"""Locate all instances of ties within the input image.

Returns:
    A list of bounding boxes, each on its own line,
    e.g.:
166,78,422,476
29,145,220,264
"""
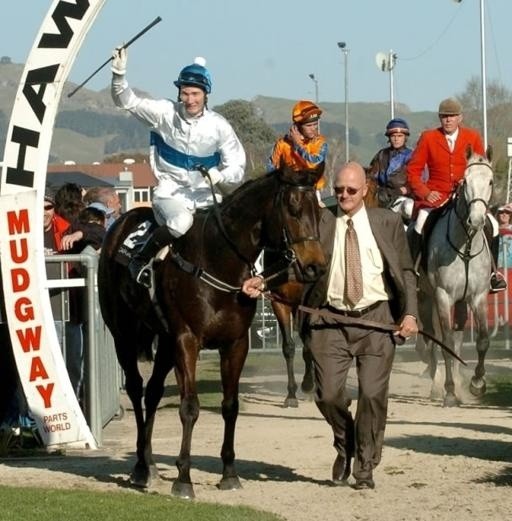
447,136,454,152
346,220,363,304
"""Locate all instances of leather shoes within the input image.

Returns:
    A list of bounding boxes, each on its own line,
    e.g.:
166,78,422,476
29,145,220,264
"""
333,455,351,482
356,479,375,490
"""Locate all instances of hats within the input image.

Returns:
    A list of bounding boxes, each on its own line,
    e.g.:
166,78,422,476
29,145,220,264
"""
88,203,114,214
45,187,55,205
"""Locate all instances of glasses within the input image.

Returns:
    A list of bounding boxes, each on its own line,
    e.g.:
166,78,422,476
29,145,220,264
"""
334,186,358,194
45,206,55,210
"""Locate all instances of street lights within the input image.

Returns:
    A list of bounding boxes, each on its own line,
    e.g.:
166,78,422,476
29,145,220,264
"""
455,0,488,148
373,47,399,124
307,71,321,135
337,41,351,162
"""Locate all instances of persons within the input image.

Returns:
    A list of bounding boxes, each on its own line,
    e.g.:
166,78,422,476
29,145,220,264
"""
10,182,121,446
241,162,417,489
367,118,427,222
270,100,327,204
407,98,506,294
496,202,512,228
111,40,246,288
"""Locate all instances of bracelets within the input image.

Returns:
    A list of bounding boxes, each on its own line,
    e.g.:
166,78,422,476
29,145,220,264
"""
405,315,416,320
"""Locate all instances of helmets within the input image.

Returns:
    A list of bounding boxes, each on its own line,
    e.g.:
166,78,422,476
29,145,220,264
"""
384,118,409,135
173,57,210,92
293,101,322,122
439,98,462,115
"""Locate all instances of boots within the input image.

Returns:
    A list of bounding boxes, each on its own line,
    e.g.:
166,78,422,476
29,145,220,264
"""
127,225,177,285
410,228,423,265
489,234,506,293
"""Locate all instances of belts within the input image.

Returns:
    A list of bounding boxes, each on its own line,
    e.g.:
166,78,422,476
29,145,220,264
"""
324,301,383,317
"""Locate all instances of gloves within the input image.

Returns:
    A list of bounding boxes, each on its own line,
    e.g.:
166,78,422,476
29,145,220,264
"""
206,168,224,185
112,40,127,75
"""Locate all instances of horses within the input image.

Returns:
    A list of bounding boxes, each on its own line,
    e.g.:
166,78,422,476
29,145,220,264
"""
348,158,437,383
408,142,497,405
98,152,325,504
262,241,317,408
